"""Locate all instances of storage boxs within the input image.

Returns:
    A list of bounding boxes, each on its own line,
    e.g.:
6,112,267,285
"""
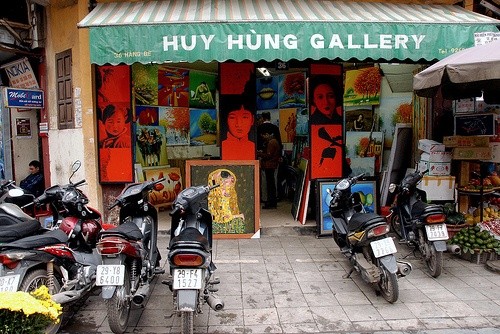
446,225,498,264
418,139,446,154
418,160,451,176
420,151,451,162
418,176,456,201
453,94,486,114
451,147,495,160
443,136,489,147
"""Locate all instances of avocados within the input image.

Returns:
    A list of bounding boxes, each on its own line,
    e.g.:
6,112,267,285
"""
447,225,500,250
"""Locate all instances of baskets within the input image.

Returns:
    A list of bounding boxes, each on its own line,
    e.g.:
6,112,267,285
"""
456,249,498,265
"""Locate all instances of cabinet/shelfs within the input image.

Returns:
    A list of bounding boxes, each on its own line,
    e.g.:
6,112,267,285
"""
457,160,500,223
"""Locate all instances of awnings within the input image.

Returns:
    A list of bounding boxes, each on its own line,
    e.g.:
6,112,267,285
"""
77,0,500,69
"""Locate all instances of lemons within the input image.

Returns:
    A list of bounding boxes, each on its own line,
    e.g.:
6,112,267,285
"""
483,207,500,222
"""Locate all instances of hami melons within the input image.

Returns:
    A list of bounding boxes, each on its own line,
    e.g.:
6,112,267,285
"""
463,207,485,224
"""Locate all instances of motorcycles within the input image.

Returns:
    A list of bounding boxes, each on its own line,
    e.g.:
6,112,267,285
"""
326,173,413,304
388,154,462,278
0,160,117,334
96,178,166,334
161,184,225,334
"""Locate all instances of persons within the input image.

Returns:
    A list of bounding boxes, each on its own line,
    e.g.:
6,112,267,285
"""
351,114,363,130
4,160,43,207
97,104,132,148
310,75,343,124
221,97,256,160
256,112,283,209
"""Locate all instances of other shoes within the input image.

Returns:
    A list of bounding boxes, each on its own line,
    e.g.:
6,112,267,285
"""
262,202,277,210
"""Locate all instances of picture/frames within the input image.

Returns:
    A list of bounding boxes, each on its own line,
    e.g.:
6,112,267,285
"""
185,158,261,240
315,177,380,240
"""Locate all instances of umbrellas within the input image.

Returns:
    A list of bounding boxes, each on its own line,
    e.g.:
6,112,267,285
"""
413,40,500,105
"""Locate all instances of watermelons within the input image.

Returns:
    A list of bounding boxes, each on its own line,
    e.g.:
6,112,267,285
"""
446,211,465,224
